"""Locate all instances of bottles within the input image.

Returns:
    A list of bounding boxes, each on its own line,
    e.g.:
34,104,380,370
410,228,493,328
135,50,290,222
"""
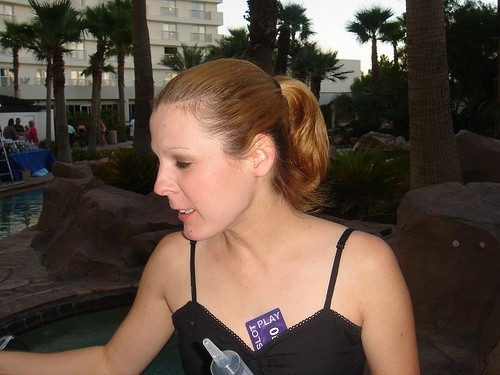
203,338,254,375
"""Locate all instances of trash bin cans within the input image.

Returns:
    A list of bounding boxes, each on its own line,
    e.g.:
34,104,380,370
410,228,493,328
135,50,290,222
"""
109,130,117,144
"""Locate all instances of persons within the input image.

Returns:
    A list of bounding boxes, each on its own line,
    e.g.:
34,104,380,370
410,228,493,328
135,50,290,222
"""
97,119,108,146
14,117,24,134
3,118,18,138
78,123,87,147
129,114,135,144
25,120,38,145
68,124,76,145
0,57,421,374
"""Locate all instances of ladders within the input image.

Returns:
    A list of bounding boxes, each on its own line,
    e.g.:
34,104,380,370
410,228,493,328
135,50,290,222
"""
0,133,14,182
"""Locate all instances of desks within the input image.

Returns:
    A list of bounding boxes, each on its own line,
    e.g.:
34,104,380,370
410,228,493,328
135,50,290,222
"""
7,148,56,180
